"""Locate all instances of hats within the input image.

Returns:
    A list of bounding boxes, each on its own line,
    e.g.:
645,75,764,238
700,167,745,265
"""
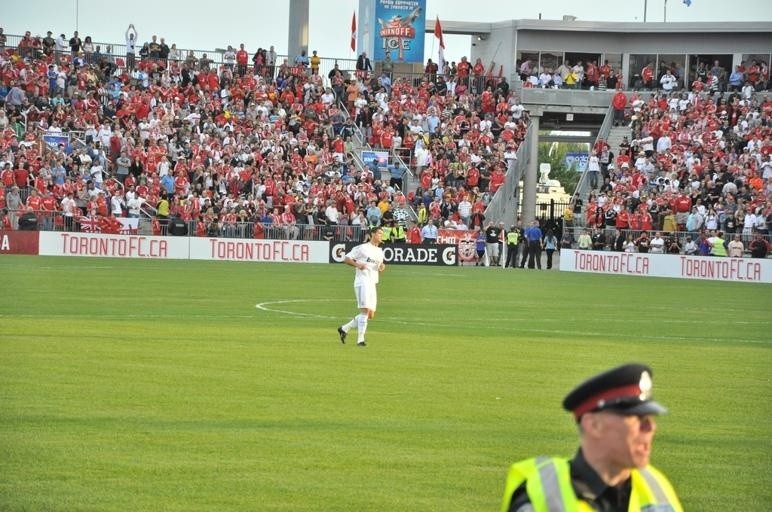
11,186,19,191
562,363,667,424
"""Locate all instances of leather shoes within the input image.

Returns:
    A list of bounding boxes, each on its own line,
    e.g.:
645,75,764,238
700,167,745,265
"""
504,264,553,269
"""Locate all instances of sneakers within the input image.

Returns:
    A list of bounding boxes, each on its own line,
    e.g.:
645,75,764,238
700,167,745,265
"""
338,326,347,344
356,342,366,346
475,261,501,267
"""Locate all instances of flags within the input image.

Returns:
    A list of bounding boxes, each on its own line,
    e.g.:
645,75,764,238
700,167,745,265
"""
434,18,445,49
351,15,356,51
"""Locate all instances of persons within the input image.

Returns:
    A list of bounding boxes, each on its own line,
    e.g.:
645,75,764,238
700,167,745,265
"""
517,58,571,89
338,227,384,346
564,59,772,258
0,27,533,245
501,363,684,511
476,216,563,268
